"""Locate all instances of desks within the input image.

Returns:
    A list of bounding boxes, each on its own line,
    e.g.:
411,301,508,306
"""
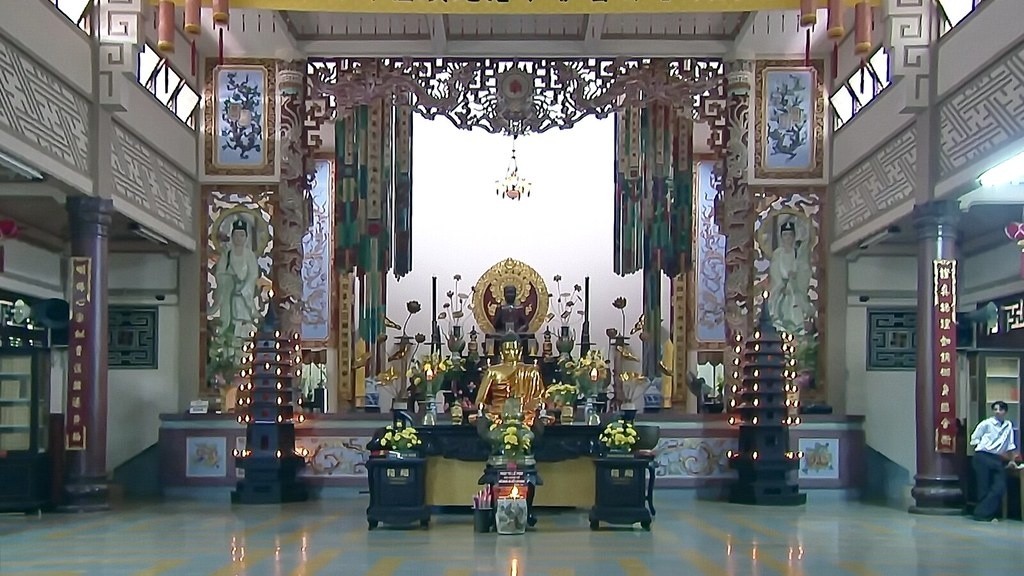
593,458,658,532
366,456,428,529
478,459,543,535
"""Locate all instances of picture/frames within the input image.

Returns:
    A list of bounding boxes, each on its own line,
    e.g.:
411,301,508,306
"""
749,57,829,187
199,55,283,183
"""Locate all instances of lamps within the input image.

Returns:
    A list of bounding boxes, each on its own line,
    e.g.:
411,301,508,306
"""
493,120,532,200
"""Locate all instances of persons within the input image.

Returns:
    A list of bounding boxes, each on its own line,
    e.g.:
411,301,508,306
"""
468,328,555,427
494,284,529,334
969,400,1018,520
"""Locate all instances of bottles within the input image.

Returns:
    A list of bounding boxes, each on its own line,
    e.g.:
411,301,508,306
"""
585,398,594,425
560,402,574,424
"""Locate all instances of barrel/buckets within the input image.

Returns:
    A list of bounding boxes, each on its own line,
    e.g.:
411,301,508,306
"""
472,507,493,533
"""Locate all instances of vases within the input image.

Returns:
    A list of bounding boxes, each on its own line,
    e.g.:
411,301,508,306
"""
557,326,573,357
448,326,465,358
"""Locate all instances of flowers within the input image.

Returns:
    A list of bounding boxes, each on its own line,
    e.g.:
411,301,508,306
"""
379,267,651,401
490,419,530,454
381,422,419,452
599,419,637,452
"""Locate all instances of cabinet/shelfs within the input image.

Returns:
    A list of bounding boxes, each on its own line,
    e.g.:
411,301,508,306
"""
0,354,50,454
985,356,1020,455
